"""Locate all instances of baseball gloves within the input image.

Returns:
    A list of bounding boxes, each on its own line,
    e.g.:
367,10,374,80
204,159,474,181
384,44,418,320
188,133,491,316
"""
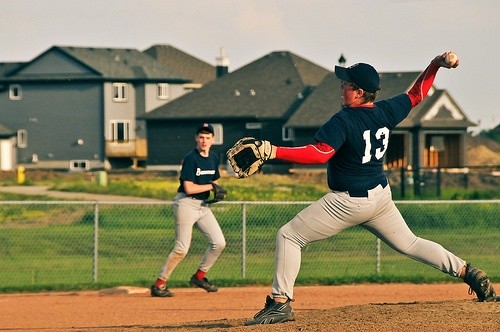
204,183,227,204
226,136,277,179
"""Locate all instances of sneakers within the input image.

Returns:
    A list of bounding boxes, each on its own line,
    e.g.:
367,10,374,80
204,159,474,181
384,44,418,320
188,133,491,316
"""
464,261,497,303
189,274,218,293
243,295,296,327
151,284,174,297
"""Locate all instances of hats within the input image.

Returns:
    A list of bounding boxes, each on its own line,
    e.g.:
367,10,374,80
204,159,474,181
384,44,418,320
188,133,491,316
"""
196,123,215,136
334,62,380,93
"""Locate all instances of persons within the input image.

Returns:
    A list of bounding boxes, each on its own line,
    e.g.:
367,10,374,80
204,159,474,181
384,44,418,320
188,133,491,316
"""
151,123,225,297
227,51,497,327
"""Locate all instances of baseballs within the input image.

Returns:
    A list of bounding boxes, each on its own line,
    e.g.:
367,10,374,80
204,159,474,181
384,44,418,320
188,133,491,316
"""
445,53,457,66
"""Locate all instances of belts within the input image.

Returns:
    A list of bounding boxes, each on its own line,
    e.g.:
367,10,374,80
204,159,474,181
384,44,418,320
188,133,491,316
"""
348,179,387,198
